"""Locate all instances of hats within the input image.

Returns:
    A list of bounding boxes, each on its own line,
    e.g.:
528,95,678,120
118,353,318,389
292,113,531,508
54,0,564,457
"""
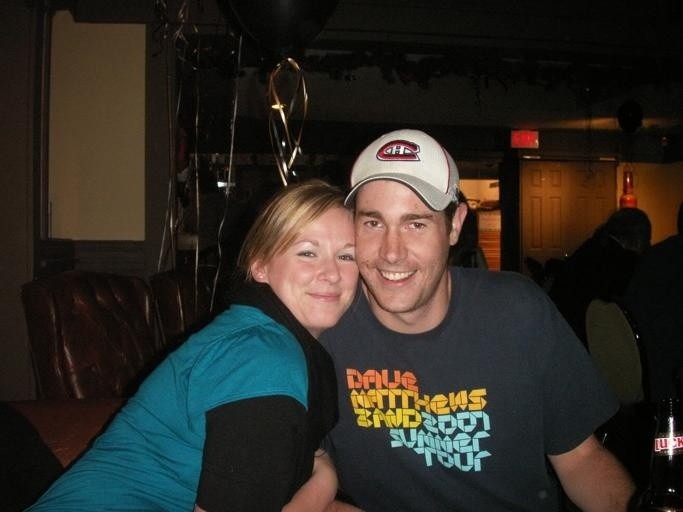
343,127,462,214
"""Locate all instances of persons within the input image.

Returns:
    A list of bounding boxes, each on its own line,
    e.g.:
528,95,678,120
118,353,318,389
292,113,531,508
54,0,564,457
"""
318,125,637,511
617,201,683,403
20,176,360,511
448,223,488,270
551,208,652,349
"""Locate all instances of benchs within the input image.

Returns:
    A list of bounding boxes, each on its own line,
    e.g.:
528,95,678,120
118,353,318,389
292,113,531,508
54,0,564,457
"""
1,397,130,512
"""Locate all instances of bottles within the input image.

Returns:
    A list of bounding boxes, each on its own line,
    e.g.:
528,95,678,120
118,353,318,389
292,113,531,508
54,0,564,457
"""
636,397,683,512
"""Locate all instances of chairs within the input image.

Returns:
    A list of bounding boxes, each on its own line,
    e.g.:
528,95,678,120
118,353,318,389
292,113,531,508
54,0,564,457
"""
16,267,165,402
151,270,208,354
